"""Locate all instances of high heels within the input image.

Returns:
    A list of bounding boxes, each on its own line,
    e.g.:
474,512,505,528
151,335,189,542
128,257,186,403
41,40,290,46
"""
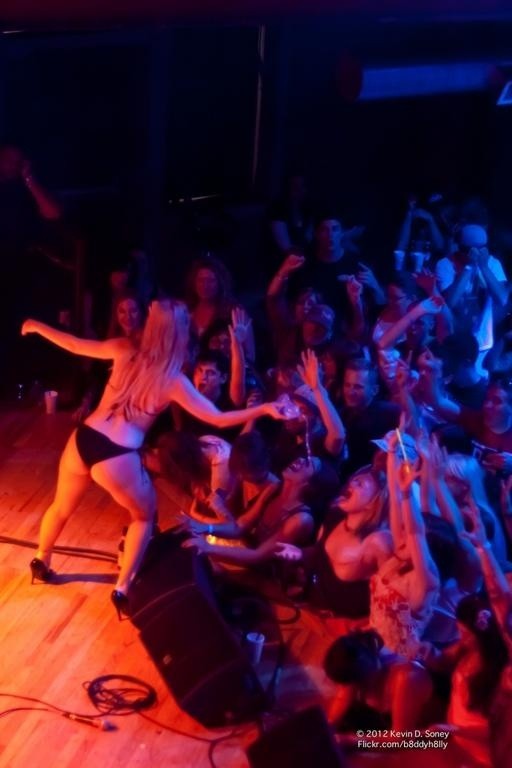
110,589,134,622
29,557,53,585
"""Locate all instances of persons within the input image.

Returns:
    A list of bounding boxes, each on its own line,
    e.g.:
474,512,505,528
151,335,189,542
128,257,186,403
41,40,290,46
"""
0,137,512,768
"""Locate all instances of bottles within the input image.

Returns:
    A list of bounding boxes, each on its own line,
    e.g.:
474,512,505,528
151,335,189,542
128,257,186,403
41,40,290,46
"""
276,394,301,421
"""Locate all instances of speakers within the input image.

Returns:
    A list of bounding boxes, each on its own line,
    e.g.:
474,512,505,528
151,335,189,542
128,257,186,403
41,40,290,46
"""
129,525,286,729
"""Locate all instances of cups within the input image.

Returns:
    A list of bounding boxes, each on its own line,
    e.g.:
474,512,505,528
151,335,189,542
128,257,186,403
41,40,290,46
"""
393,250,424,274
44,391,58,413
246,631,267,660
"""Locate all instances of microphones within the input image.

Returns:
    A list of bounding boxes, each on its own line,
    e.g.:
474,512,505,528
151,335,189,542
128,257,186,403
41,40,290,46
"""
64,712,110,731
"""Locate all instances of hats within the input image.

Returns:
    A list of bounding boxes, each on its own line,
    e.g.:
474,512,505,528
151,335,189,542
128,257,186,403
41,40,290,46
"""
455,224,488,248
370,430,418,465
303,304,335,331
